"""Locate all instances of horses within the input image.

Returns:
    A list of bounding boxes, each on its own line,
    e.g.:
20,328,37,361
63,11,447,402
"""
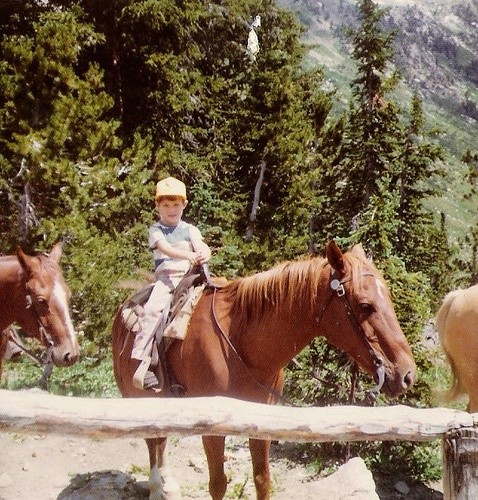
0,242,79,379
432,282,478,413
113,238,418,500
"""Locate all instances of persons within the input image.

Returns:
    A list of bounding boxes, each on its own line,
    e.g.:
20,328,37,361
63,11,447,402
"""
130,176,212,389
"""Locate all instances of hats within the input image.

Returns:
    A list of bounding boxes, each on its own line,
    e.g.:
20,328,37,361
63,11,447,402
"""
156,177,186,201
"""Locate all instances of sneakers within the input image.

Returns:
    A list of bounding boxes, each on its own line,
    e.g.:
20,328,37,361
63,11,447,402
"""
143,368,158,388
